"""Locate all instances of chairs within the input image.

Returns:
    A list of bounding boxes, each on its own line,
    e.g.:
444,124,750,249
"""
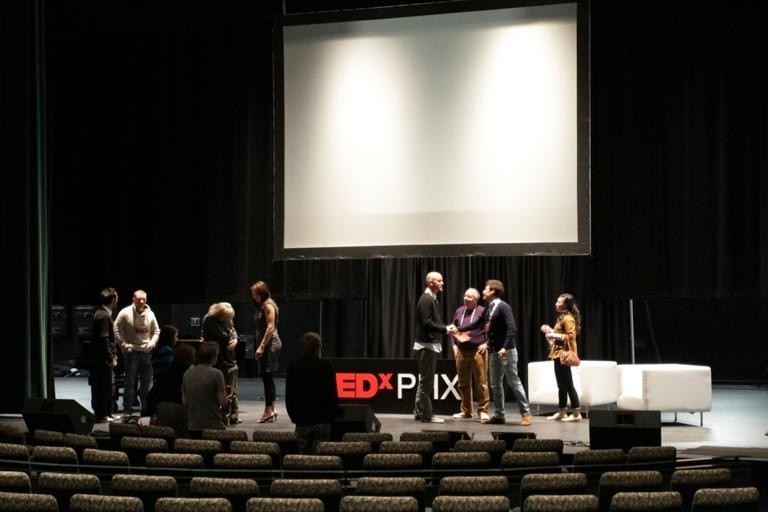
618,364,712,427
527,360,618,418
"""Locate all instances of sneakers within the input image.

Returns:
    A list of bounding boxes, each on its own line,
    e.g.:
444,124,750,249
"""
561,414,582,422
521,414,533,425
485,416,506,424
547,412,568,420
453,412,473,418
112,414,121,419
430,414,444,423
105,416,113,422
480,411,490,420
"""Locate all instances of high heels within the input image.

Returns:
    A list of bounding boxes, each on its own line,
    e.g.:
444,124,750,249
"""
256,410,278,423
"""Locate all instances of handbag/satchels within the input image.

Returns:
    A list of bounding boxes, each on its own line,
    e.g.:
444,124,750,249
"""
560,335,580,366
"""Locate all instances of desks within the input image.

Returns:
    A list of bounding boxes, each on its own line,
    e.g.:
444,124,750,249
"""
317,355,473,416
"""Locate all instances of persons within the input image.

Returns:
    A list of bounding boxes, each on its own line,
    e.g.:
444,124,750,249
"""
249,280,283,424
412,270,457,424
86,281,242,430
451,288,491,422
450,279,532,426
540,292,582,422
285,332,338,443
